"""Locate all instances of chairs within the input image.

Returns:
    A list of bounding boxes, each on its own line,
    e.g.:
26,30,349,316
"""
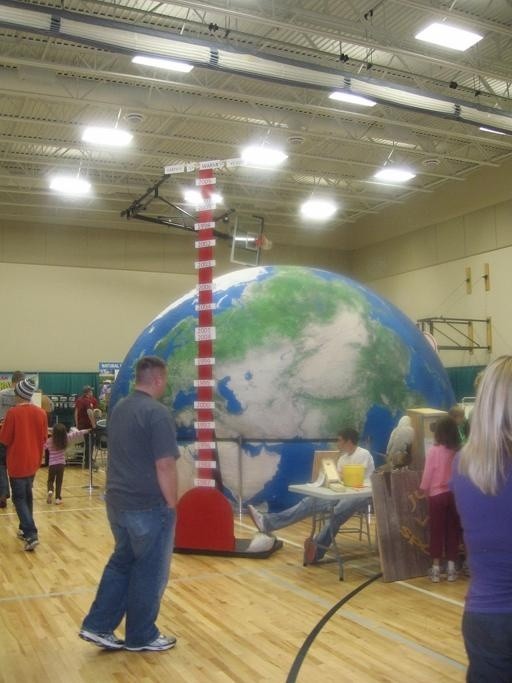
311,450,372,546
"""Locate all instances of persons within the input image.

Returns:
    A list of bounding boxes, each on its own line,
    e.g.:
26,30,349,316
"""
248,429,375,563
447,407,467,447
418,419,462,583
44,423,93,504
0,376,48,551
449,356,512,683
0,371,26,508
78,357,181,652
75,386,100,468
88,388,98,460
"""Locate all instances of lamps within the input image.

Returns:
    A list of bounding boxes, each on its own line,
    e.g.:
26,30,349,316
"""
412,17,486,52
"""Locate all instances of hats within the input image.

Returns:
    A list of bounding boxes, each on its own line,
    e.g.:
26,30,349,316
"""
85,386,94,394
15,377,35,401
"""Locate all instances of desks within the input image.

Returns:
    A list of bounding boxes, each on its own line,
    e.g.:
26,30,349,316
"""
287,480,373,581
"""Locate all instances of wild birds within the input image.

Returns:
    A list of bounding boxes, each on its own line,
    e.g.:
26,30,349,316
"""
386,416,415,470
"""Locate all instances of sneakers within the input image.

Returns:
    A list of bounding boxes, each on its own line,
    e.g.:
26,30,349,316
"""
56,498,63,504
448,568,458,582
47,490,53,504
247,505,265,532
17,529,27,541
0,496,7,507
124,634,176,651
305,539,317,563
78,624,125,650
24,537,39,551
430,567,440,583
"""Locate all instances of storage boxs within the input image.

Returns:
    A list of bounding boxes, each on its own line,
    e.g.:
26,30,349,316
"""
406,407,448,469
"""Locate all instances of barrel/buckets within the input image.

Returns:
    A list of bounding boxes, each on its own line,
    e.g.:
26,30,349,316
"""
97,419,107,450
343,464,365,486
